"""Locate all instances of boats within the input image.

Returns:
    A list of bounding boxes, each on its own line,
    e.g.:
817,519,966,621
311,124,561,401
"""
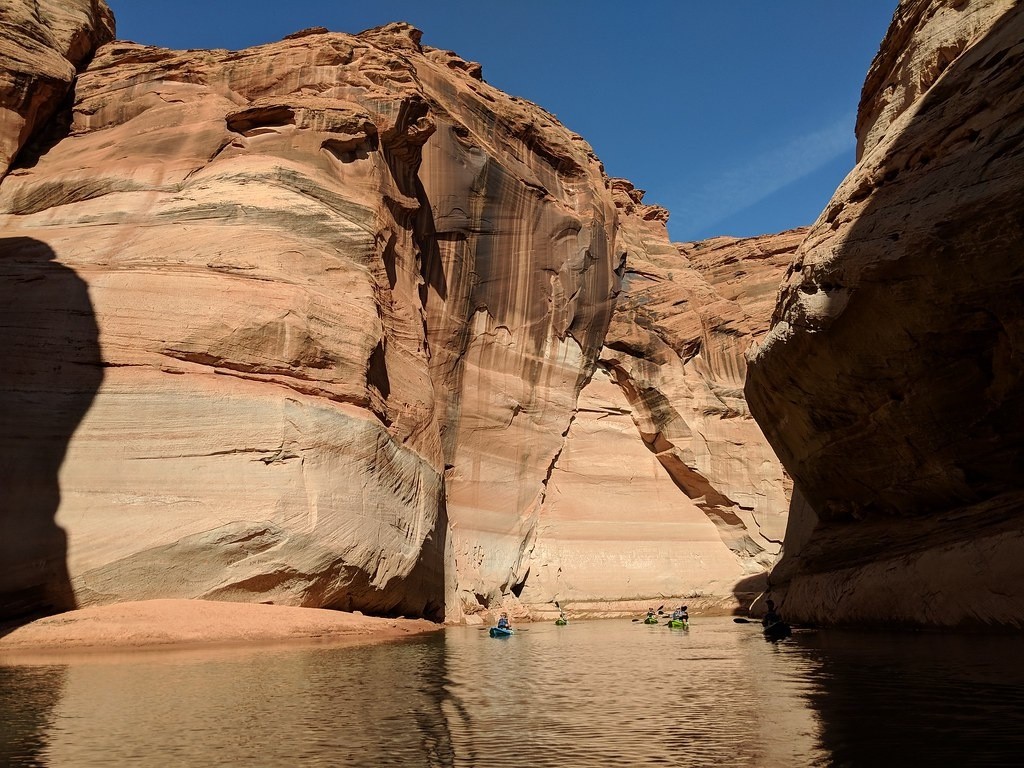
488,626,516,636
667,620,690,627
644,618,659,624
555,619,567,625
762,617,790,635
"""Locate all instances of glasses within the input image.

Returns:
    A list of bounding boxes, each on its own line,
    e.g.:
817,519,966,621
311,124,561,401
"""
500,615,505,616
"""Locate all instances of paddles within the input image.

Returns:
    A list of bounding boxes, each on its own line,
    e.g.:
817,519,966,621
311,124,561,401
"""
555,601,564,619
632,615,670,622
641,605,664,623
679,615,689,620
664,605,688,626
501,625,505,629
733,617,817,628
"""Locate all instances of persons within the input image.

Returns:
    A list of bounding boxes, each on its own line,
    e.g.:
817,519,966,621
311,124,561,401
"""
498,612,512,630
674,606,689,622
647,607,656,618
560,614,567,621
763,600,779,627
